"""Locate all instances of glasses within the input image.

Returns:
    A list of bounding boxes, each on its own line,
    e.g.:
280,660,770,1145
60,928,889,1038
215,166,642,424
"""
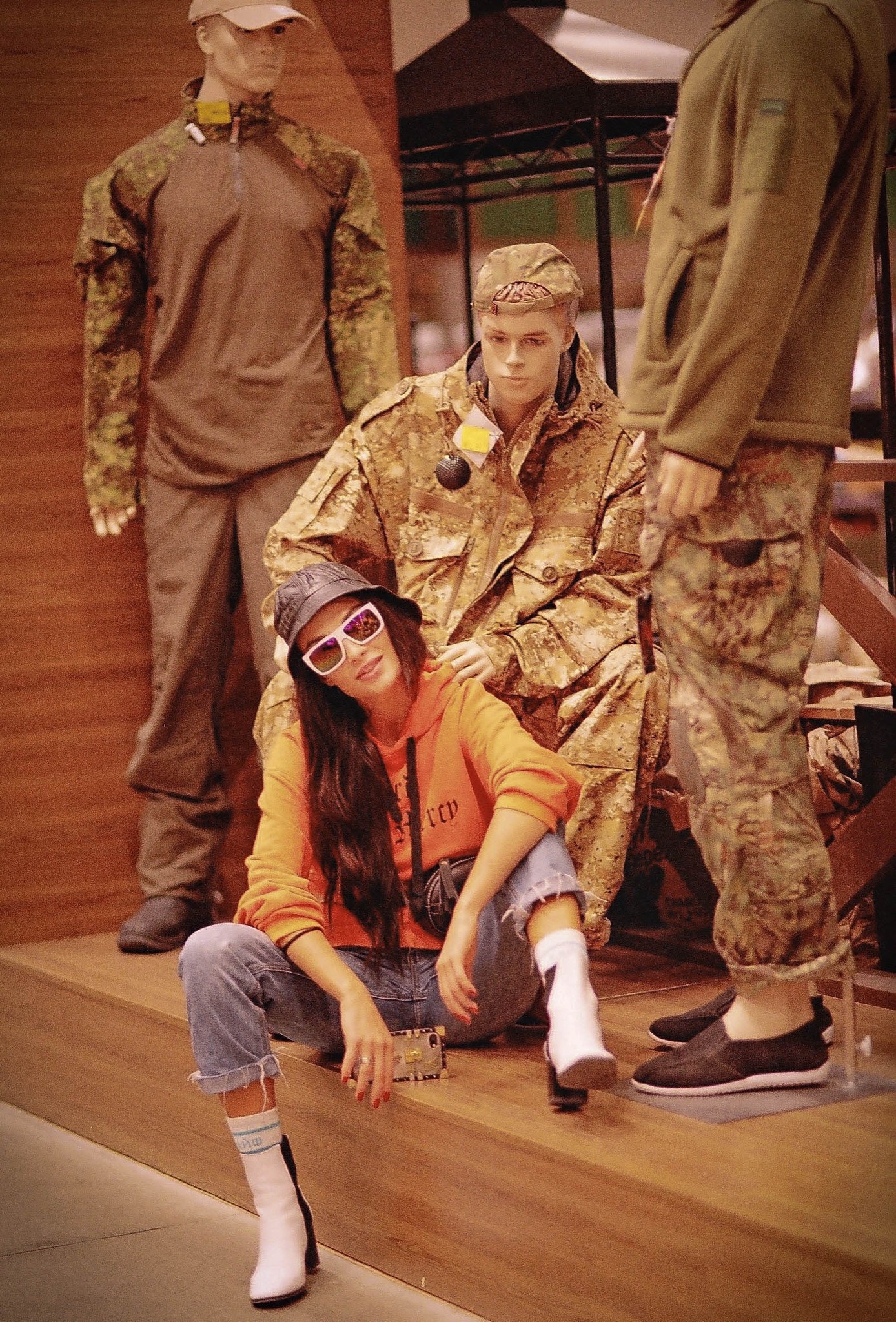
302,602,386,676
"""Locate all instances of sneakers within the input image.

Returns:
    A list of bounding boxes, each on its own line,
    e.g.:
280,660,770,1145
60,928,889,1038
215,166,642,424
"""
648,985,834,1048
117,895,216,954
632,1012,829,1096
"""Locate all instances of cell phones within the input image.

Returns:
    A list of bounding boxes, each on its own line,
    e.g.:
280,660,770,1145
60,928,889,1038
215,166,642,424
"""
350,1027,449,1083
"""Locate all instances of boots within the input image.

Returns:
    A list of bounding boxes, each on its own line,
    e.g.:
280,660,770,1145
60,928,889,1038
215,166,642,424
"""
237,1132,318,1305
541,948,617,1107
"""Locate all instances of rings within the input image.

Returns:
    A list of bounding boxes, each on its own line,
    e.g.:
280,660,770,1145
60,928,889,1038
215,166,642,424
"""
358,1057,372,1064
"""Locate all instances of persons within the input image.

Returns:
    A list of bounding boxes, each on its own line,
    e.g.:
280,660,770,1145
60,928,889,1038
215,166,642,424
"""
177,563,619,1306
617,0,896,1095
73,0,395,952
265,241,689,959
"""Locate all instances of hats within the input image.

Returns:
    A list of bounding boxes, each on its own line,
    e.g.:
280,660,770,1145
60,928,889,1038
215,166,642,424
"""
472,242,584,315
273,561,422,682
188,0,316,33
